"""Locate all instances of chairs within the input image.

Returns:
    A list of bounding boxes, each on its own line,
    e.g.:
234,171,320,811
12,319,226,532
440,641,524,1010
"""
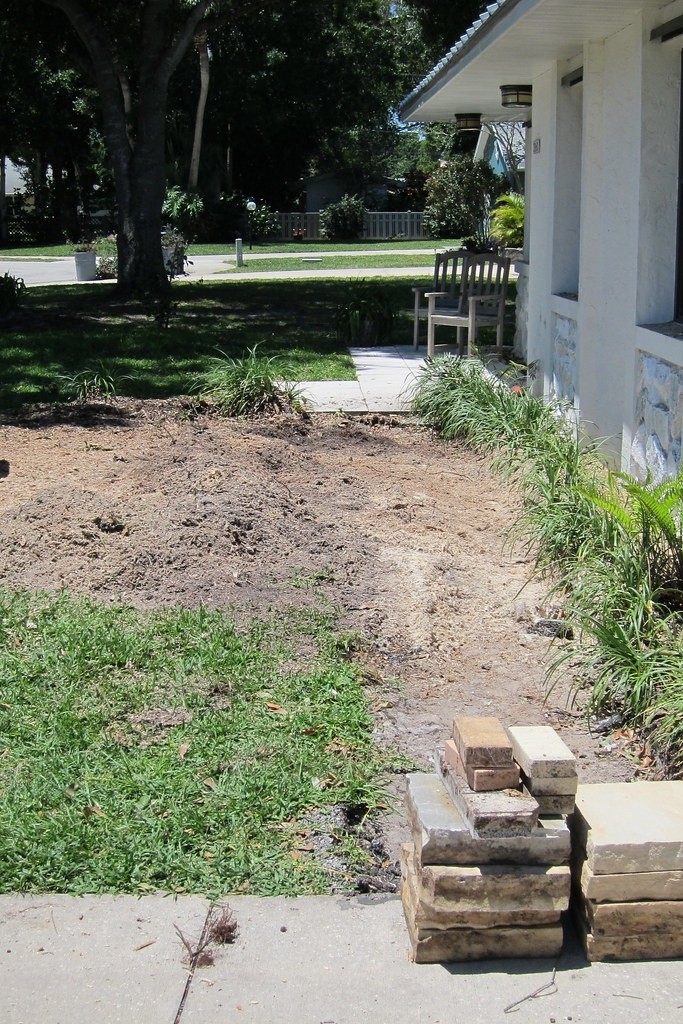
411,251,510,358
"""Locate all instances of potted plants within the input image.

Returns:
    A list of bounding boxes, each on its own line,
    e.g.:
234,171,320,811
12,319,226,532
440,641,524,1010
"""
72,236,100,281
161,223,186,273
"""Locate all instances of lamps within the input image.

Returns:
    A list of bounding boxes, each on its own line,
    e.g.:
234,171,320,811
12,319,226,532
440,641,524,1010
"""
454,114,481,130
499,84,532,108
